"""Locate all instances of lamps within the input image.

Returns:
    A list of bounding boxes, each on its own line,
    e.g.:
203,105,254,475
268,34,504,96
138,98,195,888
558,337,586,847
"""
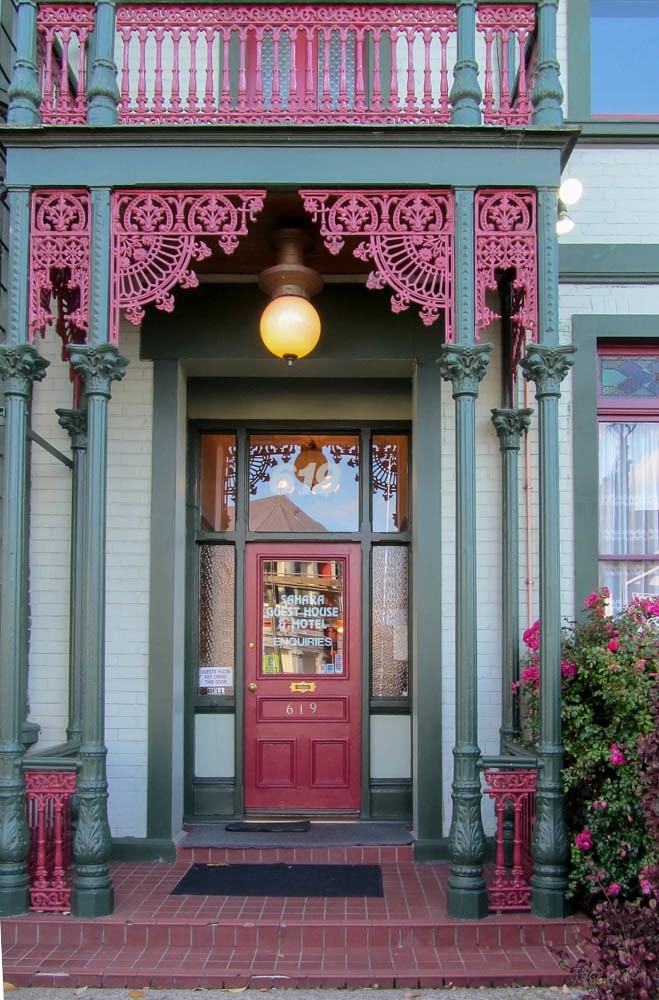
257,227,324,367
555,176,581,233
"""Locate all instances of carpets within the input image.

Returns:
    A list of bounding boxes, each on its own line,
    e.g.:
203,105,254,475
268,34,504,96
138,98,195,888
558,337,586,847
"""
169,864,385,898
182,824,416,848
